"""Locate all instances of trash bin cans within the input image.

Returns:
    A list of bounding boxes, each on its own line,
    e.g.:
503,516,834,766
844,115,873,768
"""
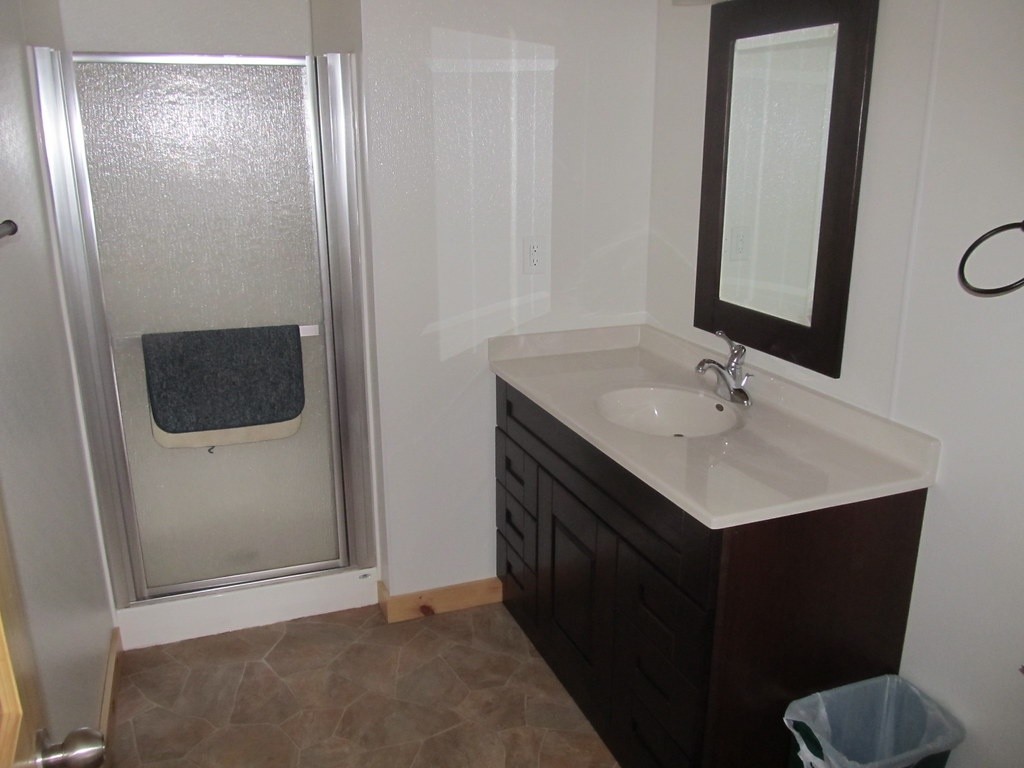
785,675,966,768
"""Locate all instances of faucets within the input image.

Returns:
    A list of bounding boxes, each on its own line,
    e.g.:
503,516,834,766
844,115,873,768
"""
694,329,754,408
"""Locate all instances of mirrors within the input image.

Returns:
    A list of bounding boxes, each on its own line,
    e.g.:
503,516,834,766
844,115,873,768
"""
694,0,882,379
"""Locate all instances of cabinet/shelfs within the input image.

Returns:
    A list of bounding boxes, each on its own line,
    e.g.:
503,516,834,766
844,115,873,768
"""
495,375,928,768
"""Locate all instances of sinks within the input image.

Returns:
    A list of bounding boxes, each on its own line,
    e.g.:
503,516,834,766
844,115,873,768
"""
595,381,747,440
